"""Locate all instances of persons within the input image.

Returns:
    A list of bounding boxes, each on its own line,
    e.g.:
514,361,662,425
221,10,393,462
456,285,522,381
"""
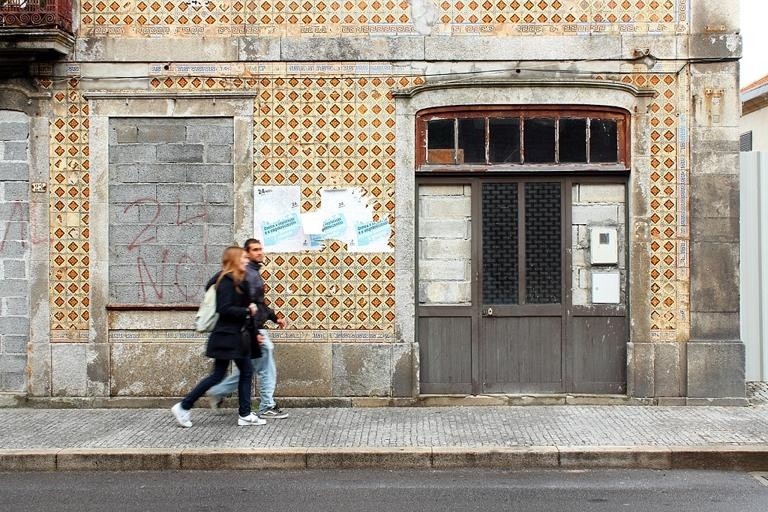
172,246,268,428
204,238,289,419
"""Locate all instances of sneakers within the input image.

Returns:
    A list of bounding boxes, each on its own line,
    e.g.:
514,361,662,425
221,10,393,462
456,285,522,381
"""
258,403,289,419
170,401,193,428
206,390,224,415
237,411,267,426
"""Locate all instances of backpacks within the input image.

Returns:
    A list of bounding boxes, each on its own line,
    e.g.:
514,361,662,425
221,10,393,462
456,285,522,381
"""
194,282,221,334
